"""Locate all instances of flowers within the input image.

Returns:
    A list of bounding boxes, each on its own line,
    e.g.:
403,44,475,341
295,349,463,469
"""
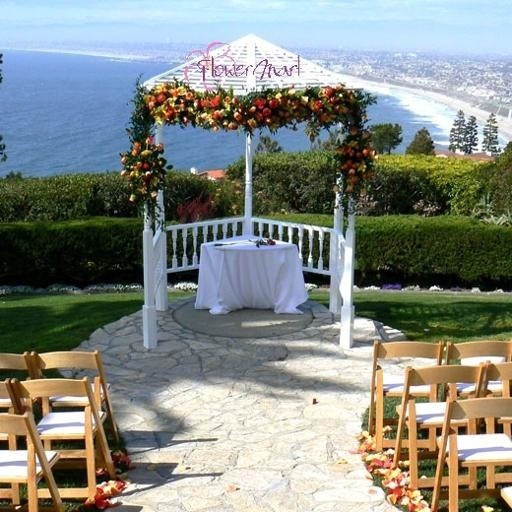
121,83,376,239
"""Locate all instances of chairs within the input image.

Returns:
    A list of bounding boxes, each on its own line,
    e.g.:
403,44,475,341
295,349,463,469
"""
0,349,123,512
368,339,512,512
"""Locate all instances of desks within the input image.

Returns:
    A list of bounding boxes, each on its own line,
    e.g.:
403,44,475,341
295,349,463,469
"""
194,234,309,315
